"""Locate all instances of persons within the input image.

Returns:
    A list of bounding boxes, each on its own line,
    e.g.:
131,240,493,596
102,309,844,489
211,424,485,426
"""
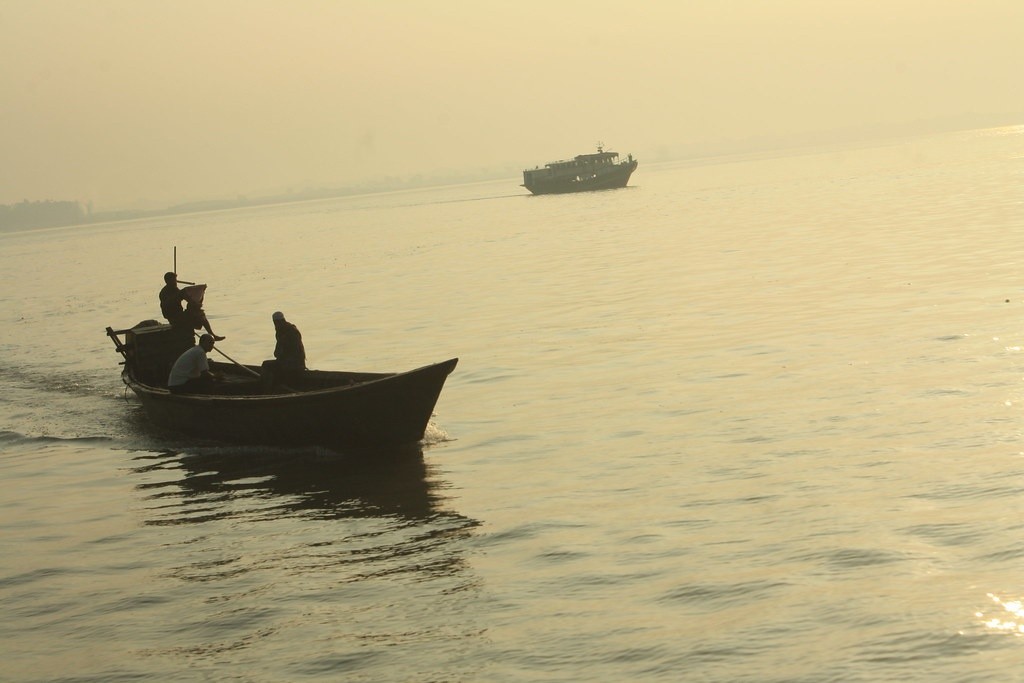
169,334,225,395
159,272,225,341
260,312,305,396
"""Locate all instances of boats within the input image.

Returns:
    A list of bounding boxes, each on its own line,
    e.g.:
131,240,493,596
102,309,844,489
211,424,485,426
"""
103,243,457,447
519,141,638,194
